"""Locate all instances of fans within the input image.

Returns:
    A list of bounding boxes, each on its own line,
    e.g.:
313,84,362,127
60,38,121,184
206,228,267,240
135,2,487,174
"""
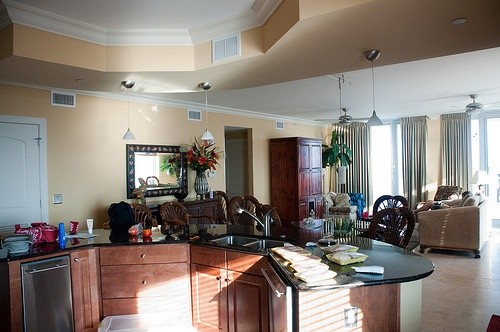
446,91,498,113
314,74,384,127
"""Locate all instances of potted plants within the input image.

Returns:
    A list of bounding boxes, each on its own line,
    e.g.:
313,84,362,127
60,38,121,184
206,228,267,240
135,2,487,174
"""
321,131,354,167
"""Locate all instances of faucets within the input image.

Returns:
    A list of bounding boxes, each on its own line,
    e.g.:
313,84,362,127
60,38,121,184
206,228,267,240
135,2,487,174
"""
235,206,276,237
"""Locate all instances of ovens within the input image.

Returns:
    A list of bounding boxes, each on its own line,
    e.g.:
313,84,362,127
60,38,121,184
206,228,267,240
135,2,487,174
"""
20,256,75,332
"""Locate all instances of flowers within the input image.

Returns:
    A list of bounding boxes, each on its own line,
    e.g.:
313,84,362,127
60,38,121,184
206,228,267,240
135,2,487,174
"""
185,141,224,174
160,153,182,182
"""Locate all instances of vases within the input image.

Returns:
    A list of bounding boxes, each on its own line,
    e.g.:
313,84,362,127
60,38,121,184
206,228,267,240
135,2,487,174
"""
193,169,210,194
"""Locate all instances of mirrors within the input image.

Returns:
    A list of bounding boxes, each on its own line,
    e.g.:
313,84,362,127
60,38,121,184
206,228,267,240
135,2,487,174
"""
126,144,188,198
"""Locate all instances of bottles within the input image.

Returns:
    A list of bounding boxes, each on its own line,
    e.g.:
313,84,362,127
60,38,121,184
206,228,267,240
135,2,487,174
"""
58,223,66,249
309,209,315,220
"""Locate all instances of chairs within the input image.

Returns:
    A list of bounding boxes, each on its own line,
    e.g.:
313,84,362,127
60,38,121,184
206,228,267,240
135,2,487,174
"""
434,186,464,202
363,196,416,248
204,190,281,238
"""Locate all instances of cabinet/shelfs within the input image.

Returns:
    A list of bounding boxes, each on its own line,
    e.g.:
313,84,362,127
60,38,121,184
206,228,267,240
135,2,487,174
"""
266,260,288,332
194,246,269,332
135,200,221,230
101,243,191,316
70,248,103,332
269,136,322,238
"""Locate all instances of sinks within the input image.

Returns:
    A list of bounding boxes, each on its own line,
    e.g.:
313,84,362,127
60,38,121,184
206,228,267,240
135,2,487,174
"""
246,240,288,249
209,235,257,246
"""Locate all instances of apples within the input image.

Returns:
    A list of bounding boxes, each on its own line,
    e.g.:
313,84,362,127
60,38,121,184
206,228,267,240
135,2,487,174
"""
128,228,137,235
142,229,152,237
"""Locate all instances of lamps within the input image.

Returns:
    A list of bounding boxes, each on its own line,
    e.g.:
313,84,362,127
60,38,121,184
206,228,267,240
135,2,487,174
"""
469,170,492,195
338,165,347,185
357,50,385,126
120,81,136,139
201,83,213,140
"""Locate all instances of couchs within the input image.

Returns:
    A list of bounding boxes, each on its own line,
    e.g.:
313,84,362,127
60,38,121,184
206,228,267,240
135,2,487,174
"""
322,191,359,219
418,198,492,259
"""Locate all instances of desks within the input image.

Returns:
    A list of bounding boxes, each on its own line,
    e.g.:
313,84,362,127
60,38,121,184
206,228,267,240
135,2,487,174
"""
293,214,373,235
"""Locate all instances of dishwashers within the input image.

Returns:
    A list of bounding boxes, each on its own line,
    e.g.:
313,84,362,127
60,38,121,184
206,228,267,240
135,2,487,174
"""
261,260,294,332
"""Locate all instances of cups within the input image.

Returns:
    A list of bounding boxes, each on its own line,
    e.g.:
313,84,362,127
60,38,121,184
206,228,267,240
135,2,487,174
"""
69,221,79,235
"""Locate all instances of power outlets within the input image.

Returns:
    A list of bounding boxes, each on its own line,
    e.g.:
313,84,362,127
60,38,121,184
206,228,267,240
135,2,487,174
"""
345,307,359,325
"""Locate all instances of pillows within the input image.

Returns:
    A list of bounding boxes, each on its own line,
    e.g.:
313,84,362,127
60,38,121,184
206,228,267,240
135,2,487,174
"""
459,194,480,206
